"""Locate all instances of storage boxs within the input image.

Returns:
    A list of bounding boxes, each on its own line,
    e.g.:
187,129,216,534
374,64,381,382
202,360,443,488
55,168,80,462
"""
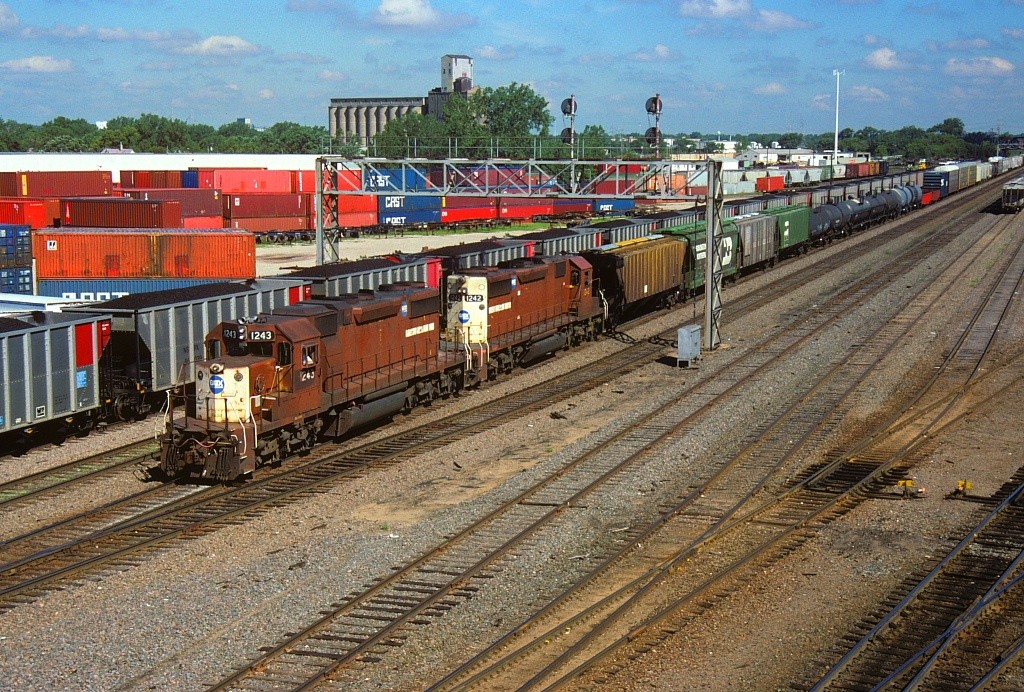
0,160,987,431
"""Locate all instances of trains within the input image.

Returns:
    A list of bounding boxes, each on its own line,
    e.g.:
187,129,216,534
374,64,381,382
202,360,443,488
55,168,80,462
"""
0,155,1024,482
1001,173,1024,214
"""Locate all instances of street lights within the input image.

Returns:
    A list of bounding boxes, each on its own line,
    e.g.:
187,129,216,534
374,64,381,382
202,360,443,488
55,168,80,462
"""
833,67,846,165
